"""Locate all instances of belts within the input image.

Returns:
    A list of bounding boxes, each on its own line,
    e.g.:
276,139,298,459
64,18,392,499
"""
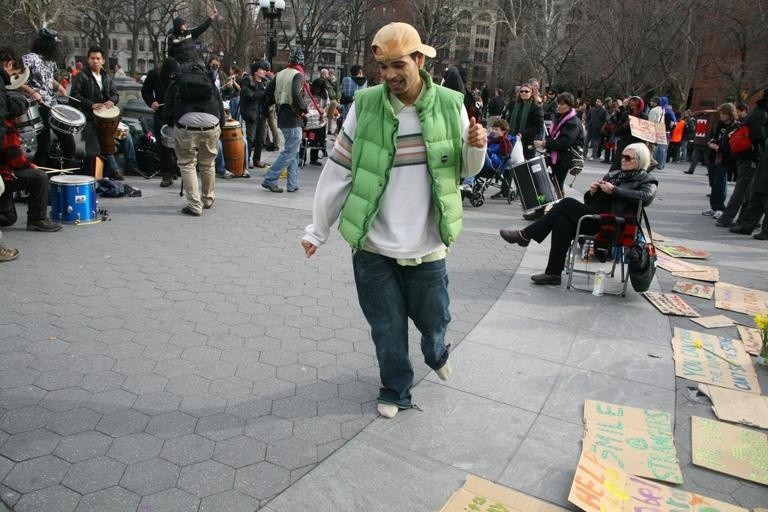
175,120,220,131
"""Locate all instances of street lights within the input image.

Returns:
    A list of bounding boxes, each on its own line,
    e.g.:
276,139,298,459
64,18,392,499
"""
259,0,284,76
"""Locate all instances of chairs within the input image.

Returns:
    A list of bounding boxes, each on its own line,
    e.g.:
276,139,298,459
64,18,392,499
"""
559,196,645,300
299,128,328,168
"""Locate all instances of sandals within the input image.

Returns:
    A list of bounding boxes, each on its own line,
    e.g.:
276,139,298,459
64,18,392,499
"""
492,192,508,200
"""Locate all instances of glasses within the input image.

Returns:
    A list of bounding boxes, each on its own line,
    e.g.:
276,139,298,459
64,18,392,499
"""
520,90,531,93
619,154,636,161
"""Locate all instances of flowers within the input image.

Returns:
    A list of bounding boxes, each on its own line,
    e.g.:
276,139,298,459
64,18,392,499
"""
751,308,768,357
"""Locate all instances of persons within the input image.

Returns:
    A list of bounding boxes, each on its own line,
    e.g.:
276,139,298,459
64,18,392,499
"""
701,89,768,240
302,19,490,418
106,132,140,180
500,142,659,285
1,27,119,260
310,65,368,165
260,51,305,192
440,68,586,199
141,8,275,217
586,96,675,172
666,109,710,175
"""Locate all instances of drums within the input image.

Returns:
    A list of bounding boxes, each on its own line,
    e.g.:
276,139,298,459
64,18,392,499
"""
114,122,131,141
49,173,97,225
220,120,245,178
17,98,45,136
302,110,327,149
159,123,176,149
92,104,120,155
47,104,88,135
506,156,563,214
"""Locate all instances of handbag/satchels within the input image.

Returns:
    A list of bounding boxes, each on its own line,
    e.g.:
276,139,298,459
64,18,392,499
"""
629,243,658,292
569,145,584,187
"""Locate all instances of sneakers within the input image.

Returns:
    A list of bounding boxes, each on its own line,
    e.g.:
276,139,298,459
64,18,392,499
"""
1,246,19,263
531,272,561,285
702,208,768,246
26,211,62,232
459,183,473,194
523,209,544,220
584,156,615,164
108,157,322,215
500,229,530,246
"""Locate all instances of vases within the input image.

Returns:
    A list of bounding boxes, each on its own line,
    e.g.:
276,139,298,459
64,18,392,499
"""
758,353,768,368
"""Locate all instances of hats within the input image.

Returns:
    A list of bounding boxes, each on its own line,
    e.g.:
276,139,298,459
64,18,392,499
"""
370,21,437,62
649,96,661,104
39,28,62,44
1,91,29,119
288,48,304,66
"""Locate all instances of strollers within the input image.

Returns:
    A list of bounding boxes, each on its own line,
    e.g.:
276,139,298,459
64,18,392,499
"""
456,134,517,204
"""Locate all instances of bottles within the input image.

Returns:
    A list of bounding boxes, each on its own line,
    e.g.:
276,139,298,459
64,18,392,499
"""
559,239,605,299
